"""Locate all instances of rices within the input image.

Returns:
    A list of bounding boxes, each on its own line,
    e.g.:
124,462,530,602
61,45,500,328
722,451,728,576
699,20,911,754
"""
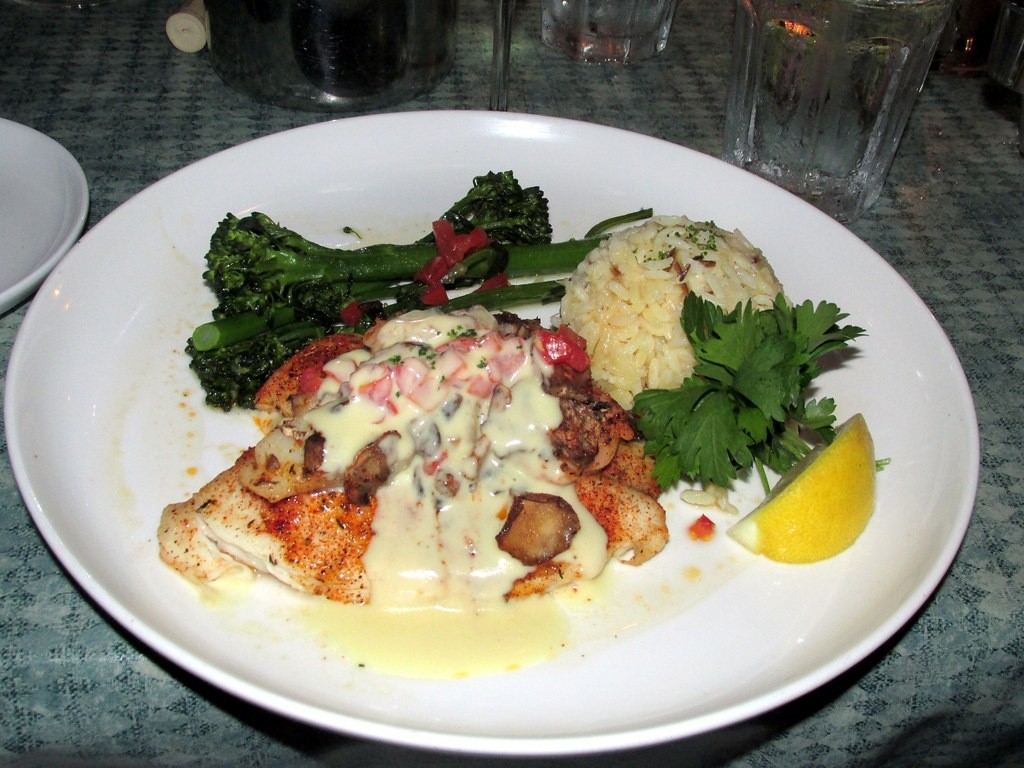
550,215,792,515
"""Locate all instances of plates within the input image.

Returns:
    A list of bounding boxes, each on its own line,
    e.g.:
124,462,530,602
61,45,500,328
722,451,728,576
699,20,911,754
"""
0,118,89,314
3,112,979,754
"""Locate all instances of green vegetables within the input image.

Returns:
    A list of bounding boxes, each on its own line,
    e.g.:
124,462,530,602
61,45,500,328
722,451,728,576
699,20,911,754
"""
631,291,891,500
185,168,655,411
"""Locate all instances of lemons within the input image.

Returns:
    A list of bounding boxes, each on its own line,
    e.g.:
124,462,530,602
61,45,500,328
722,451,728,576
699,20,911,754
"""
728,413,875,563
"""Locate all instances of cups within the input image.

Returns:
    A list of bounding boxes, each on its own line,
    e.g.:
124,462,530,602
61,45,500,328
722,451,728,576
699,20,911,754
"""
203,0,459,114
987,0,1024,95
722,0,955,225
542,0,677,62
